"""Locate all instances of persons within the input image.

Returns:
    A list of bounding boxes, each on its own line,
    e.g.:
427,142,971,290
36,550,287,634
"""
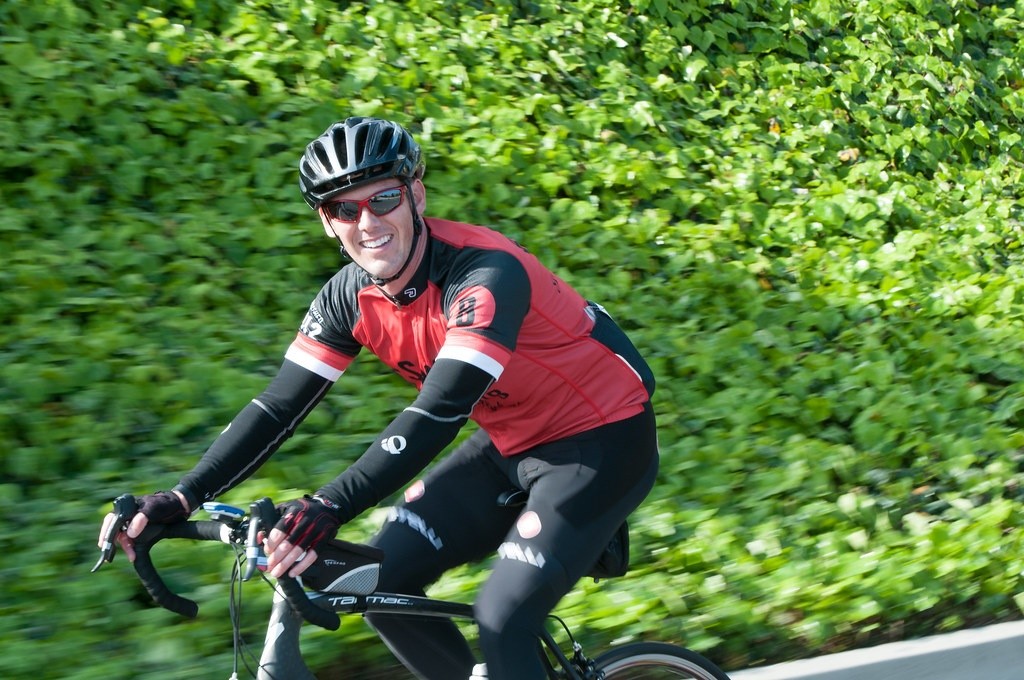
98,116,660,680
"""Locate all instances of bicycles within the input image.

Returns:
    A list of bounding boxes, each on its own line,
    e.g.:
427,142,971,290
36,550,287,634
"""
85,495,735,680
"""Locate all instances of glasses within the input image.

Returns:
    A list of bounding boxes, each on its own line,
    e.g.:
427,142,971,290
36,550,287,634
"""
318,178,417,223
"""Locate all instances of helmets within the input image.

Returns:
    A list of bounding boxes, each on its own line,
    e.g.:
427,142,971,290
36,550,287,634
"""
297,117,426,212
